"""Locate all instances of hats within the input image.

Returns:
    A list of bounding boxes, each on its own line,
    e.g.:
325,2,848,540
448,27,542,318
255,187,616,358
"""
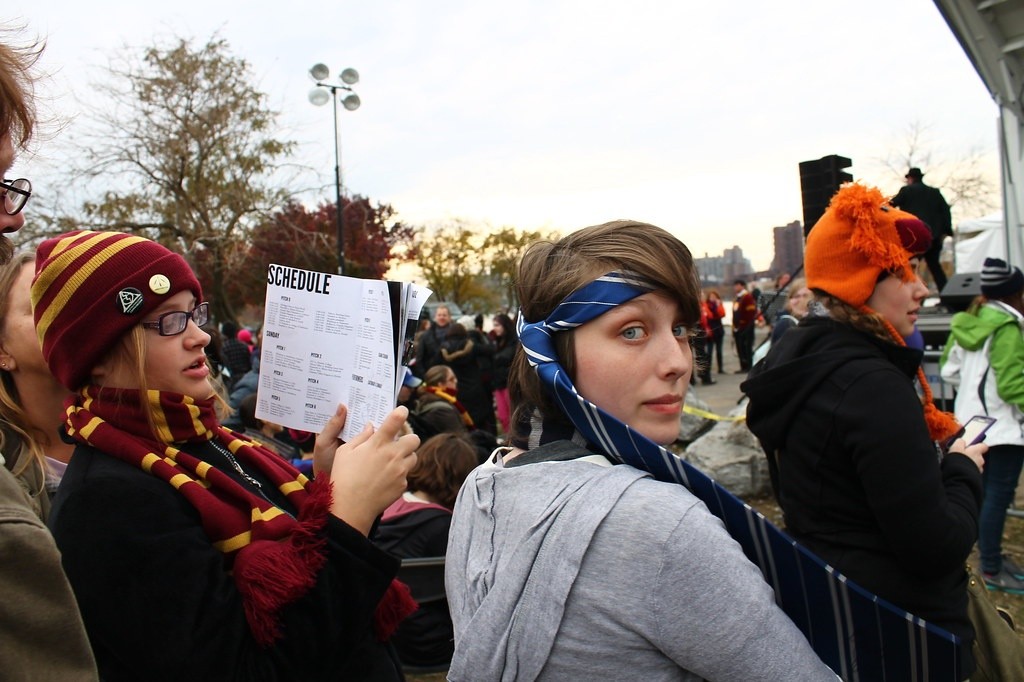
402,366,422,388
221,321,237,339
905,168,924,178
238,330,251,343
30,230,204,392
804,183,962,441
980,258,1024,300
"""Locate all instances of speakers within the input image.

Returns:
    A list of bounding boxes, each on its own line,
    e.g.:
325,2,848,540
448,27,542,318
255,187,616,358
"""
940,272,984,313
798,154,853,236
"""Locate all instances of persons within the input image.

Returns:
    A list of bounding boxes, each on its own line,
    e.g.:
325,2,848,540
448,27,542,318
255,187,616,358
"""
766,277,815,348
688,288,725,386
887,168,954,308
750,282,761,302
0,253,77,528
371,432,480,666
939,255,1024,596
30,230,421,682
732,279,757,373
775,273,792,290
199,308,519,511
740,183,989,682
445,220,842,682
0,25,99,682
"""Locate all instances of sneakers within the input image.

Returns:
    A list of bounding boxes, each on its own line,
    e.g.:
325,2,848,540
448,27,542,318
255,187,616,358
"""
983,554,1024,593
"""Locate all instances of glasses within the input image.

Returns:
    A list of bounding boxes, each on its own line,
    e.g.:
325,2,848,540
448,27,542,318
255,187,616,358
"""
143,301,211,336
0,177,32,216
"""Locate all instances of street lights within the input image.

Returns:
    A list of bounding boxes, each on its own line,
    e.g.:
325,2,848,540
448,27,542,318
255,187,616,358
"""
308,62,369,276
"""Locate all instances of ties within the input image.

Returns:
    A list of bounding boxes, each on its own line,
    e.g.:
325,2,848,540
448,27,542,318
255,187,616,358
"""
515,266,956,682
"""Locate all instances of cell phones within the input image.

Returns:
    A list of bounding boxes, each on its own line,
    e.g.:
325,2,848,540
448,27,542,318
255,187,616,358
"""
945,415,997,451
